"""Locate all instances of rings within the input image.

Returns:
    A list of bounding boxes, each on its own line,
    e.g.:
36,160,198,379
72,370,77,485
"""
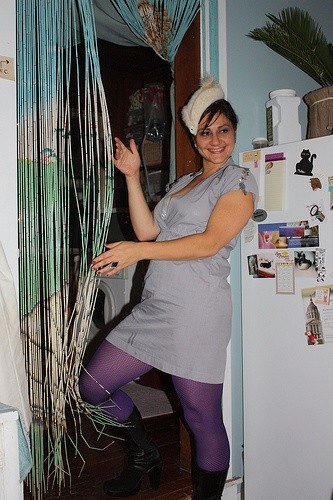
110,261,119,268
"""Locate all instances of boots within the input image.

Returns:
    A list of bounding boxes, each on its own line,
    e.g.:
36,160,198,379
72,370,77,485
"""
191,466,230,500
104,404,162,496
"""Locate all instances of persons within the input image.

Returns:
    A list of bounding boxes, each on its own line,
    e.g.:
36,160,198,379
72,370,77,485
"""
77,76,261,500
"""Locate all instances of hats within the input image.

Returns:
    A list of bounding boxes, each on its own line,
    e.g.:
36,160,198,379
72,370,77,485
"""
182,75,224,135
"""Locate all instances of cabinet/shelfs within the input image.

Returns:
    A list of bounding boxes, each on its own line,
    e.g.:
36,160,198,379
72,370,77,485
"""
65,13,203,473
